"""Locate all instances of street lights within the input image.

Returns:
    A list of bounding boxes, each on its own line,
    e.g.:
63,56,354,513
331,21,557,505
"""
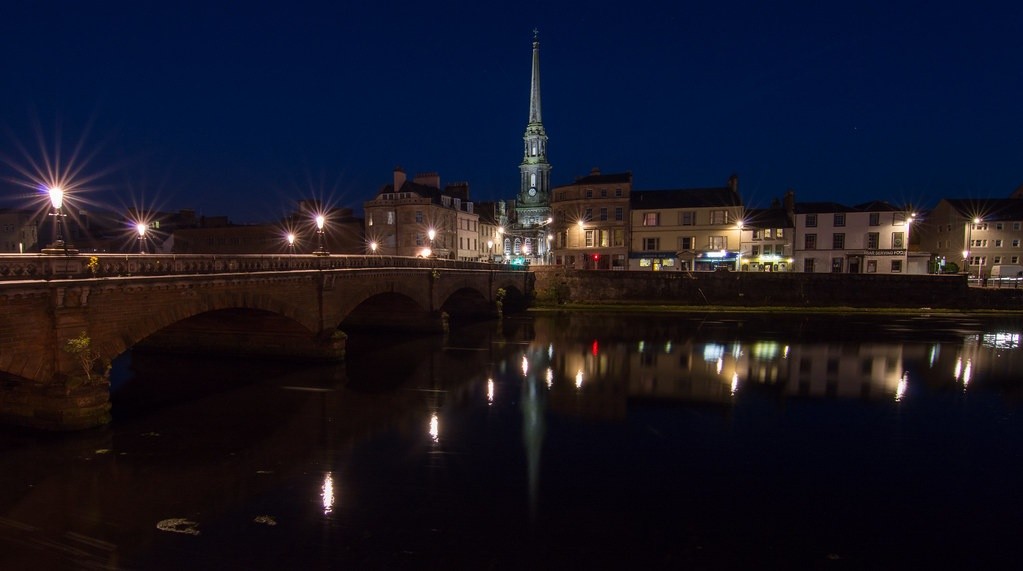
429,226,436,258
371,238,377,255
136,220,147,254
313,212,329,254
286,231,294,254
964,213,981,273
488,241,494,264
735,219,742,272
48,185,71,256
903,209,915,271
